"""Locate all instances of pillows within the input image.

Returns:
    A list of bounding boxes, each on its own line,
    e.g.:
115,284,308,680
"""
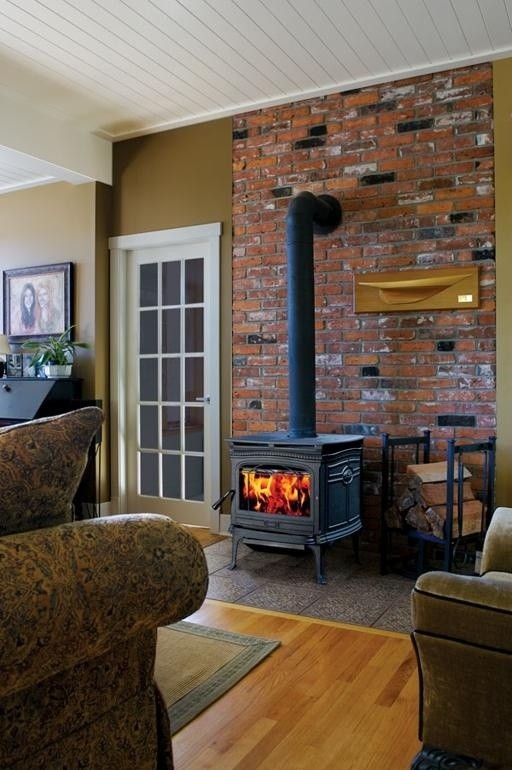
1,406,105,536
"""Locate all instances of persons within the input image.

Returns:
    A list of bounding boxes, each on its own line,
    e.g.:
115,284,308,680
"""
12,282,62,336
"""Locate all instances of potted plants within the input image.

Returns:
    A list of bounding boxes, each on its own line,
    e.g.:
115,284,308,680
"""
21,323,90,378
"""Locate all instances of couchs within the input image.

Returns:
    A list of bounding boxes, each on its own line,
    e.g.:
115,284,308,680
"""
1,403,212,770
408,505,512,770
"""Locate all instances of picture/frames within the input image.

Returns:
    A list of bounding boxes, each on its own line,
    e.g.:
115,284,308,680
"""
2,263,75,343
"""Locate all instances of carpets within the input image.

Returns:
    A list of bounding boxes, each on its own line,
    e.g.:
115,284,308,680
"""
155,619,282,743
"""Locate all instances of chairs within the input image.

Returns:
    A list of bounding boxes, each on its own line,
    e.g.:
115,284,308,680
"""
408,434,499,573
384,431,433,568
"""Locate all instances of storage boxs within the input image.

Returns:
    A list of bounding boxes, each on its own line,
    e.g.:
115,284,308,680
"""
402,457,477,509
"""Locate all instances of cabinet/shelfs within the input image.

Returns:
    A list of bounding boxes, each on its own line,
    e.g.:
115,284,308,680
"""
0,374,87,509
